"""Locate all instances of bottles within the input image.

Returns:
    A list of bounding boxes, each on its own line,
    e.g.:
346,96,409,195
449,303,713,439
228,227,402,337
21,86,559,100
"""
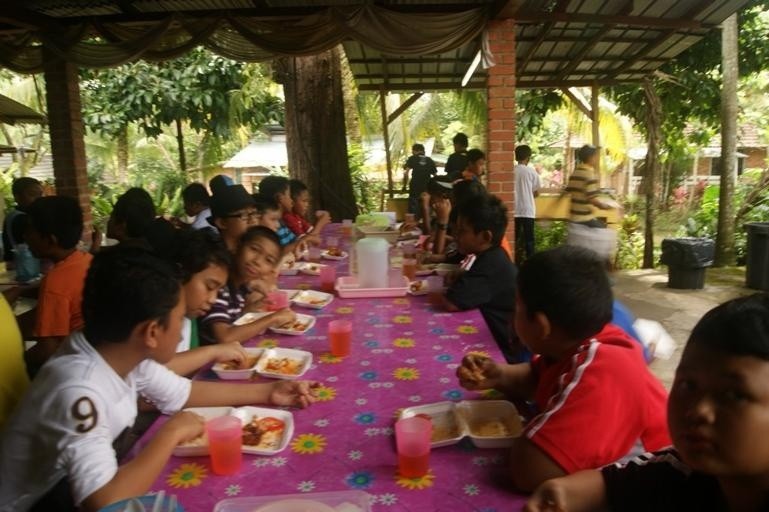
16,243,37,277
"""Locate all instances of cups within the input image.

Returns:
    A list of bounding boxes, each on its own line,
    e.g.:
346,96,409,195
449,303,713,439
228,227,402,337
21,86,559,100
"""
403,258,417,281
342,218,353,237
271,289,290,305
329,319,353,358
308,247,320,264
326,236,338,254
318,265,336,293
394,417,434,481
205,417,245,477
404,212,414,225
402,244,416,258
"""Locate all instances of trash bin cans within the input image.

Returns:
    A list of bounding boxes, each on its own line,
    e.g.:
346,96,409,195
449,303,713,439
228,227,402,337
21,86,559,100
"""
661,237,713,289
742,222,769,290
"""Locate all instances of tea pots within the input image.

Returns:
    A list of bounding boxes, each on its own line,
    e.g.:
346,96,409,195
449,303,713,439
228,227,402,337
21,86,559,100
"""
348,235,393,288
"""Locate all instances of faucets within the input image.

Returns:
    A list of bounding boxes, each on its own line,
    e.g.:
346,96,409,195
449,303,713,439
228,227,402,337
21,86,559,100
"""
548,178,563,189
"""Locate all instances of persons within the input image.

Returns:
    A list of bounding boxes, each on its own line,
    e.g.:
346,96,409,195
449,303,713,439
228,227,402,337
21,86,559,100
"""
106,175,331,438
0,245,323,511
525,292,768,512
0,294,29,425
456,245,673,491
549,161,562,192
401,134,525,364
513,145,540,265
0,177,94,378
568,145,615,273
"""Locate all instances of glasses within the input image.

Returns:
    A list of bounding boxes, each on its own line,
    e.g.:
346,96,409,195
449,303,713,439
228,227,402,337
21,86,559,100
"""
218,210,262,221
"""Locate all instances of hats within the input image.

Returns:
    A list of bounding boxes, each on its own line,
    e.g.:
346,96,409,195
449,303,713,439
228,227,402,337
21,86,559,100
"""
577,144,602,160
205,184,265,226
